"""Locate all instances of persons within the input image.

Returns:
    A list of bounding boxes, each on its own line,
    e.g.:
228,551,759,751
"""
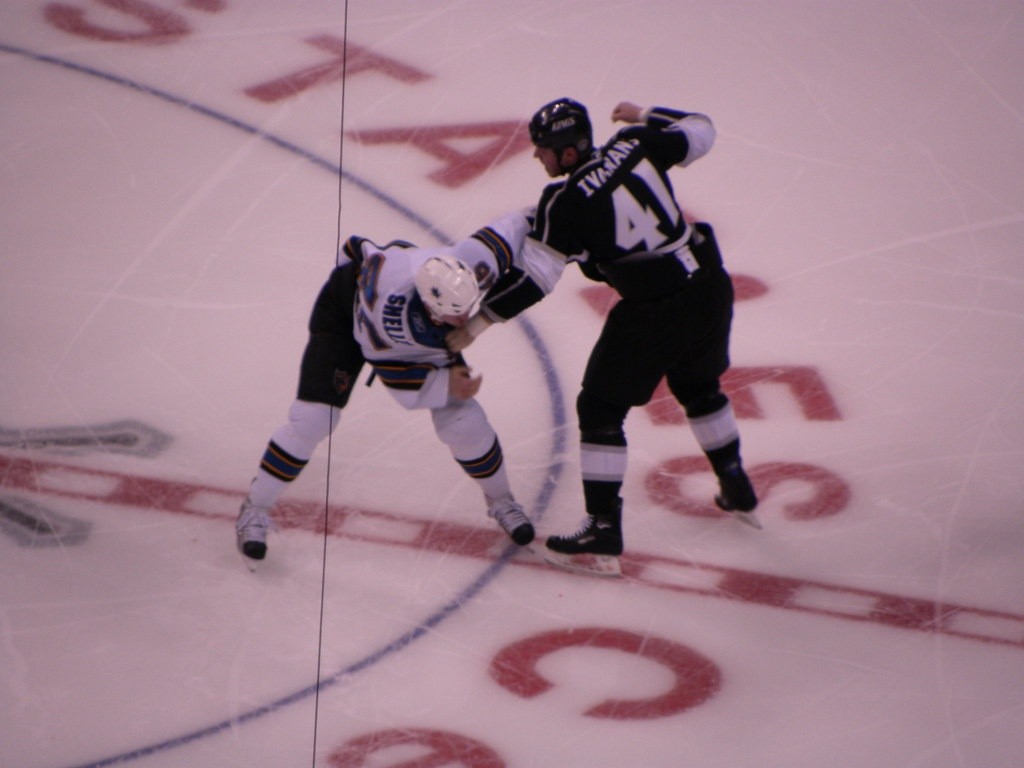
235,208,537,572
447,98,760,572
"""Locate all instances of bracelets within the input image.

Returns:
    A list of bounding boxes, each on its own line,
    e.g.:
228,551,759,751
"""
639,109,645,121
465,314,490,337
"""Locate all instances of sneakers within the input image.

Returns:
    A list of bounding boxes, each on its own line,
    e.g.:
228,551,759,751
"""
546,496,624,576
482,486,530,553
235,472,291,572
716,460,764,528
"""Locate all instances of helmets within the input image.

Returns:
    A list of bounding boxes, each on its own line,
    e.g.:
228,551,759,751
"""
529,96,591,153
415,256,481,320
